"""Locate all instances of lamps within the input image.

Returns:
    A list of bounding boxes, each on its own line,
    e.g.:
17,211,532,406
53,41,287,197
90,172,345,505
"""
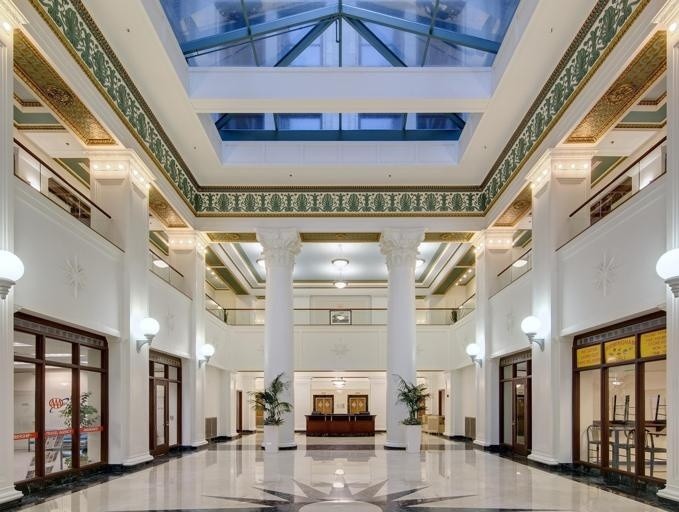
136,318,160,353
656,249,679,297
466,343,482,368
198,344,215,368
332,273,347,288
1,250,24,300
415,256,425,268
521,316,544,351
256,251,265,266
331,245,349,267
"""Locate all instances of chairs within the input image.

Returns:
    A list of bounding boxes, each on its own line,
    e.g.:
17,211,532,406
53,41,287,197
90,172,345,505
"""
422,414,445,435
586,394,667,478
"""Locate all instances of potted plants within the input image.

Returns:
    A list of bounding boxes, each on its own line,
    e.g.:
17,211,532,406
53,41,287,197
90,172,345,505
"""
391,373,432,455
247,372,295,454
59,392,101,469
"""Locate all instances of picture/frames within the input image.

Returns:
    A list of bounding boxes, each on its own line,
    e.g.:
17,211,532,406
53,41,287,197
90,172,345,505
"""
329,309,352,324
